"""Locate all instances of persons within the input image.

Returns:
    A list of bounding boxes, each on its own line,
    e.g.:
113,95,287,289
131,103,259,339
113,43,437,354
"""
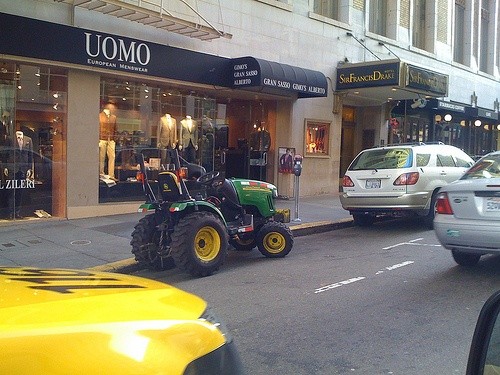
4,131,34,219
157,113,179,170
179,115,199,165
280,148,292,172
98,103,118,179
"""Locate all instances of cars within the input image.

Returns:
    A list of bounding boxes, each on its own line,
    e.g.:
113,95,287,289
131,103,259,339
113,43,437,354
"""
433,150,500,267
0,266,247,375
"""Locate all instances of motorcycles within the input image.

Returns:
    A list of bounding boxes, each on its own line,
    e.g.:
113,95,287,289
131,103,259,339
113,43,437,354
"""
129,170,293,276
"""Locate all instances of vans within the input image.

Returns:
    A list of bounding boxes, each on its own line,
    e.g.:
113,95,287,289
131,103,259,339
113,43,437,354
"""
339,142,478,229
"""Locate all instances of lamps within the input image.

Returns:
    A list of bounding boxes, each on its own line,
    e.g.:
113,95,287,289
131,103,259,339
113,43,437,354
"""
443,113,500,132
